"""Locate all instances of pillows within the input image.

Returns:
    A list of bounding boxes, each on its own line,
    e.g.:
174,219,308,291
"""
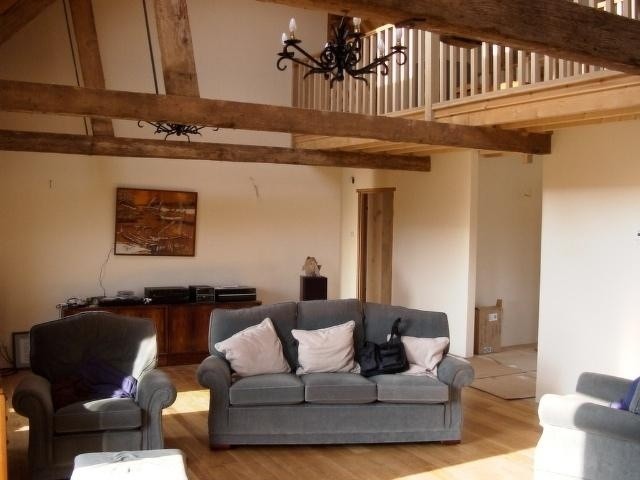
213,318,291,377
291,320,361,375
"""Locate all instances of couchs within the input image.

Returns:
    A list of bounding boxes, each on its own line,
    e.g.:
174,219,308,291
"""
534,372,640,479
198,299,474,449
13,310,177,480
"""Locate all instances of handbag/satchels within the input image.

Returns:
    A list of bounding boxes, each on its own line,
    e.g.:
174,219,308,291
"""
357,317,409,377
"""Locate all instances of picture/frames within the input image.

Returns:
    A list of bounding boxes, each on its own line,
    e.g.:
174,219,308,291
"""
115,187,198,257
12,333,30,370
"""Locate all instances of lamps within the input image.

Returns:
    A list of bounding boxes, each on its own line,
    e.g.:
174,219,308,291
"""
274,8,406,88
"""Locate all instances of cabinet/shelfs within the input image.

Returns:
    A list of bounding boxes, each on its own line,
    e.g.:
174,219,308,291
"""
63,302,261,367
